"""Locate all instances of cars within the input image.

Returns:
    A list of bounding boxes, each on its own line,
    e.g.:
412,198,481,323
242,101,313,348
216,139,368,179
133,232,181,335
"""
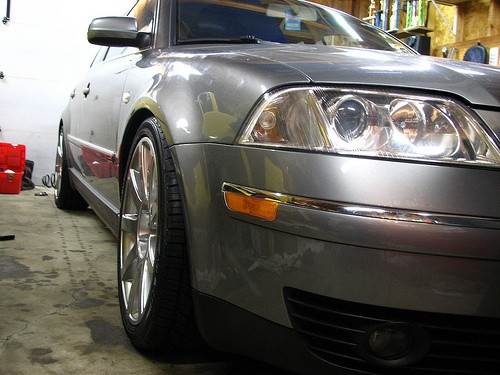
53,0,500,375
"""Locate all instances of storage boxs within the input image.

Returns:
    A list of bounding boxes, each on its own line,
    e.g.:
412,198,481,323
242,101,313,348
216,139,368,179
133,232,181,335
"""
0,142,26,194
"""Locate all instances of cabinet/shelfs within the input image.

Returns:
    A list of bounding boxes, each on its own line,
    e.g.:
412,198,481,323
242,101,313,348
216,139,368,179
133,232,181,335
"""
359,0,435,57
